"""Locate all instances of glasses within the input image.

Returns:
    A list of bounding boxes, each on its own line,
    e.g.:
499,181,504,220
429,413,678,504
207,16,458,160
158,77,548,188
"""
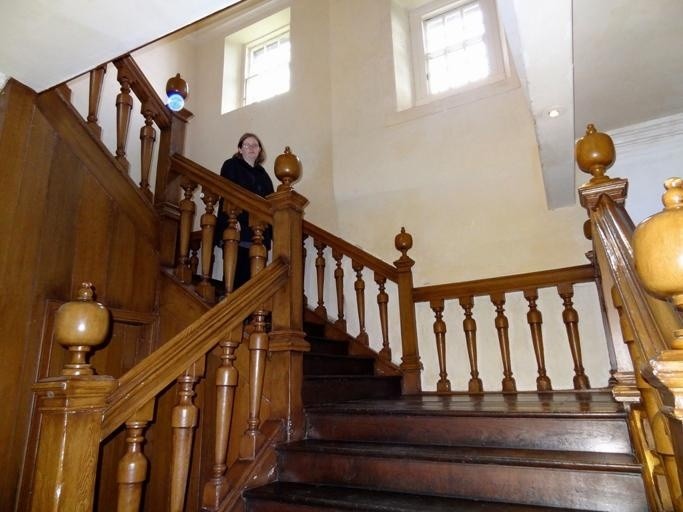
243,143,260,150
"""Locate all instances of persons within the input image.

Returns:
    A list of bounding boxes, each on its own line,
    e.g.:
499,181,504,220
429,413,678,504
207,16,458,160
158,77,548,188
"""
210,133,276,296
